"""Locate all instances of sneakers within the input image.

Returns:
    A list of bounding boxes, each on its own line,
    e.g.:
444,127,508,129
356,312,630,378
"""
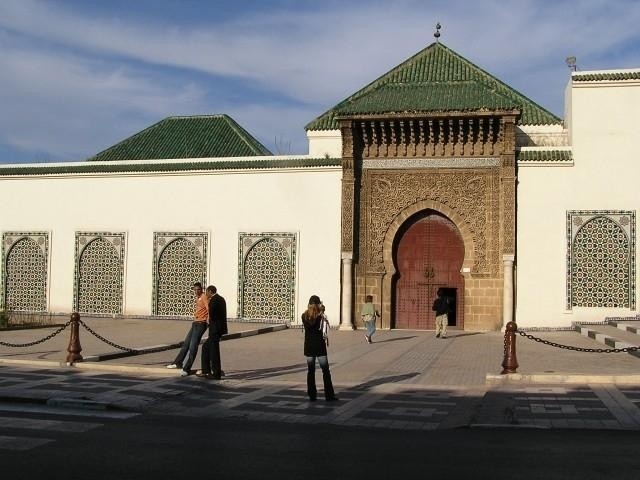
181,371,188,376
167,364,177,369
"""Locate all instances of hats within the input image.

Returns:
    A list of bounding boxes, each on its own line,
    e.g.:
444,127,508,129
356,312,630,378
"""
309,295,321,304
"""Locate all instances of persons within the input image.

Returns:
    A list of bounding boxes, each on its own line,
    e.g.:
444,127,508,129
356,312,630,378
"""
168,282,207,378
195,286,229,381
301,295,340,404
431,290,450,340
361,294,381,345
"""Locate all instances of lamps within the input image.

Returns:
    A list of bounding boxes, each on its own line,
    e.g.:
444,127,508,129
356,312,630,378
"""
565,54,579,73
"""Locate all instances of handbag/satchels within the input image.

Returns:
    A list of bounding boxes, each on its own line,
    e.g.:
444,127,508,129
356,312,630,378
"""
319,315,329,338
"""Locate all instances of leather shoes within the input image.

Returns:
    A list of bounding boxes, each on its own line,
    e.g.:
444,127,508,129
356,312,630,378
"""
196,373,212,377
206,374,220,380
